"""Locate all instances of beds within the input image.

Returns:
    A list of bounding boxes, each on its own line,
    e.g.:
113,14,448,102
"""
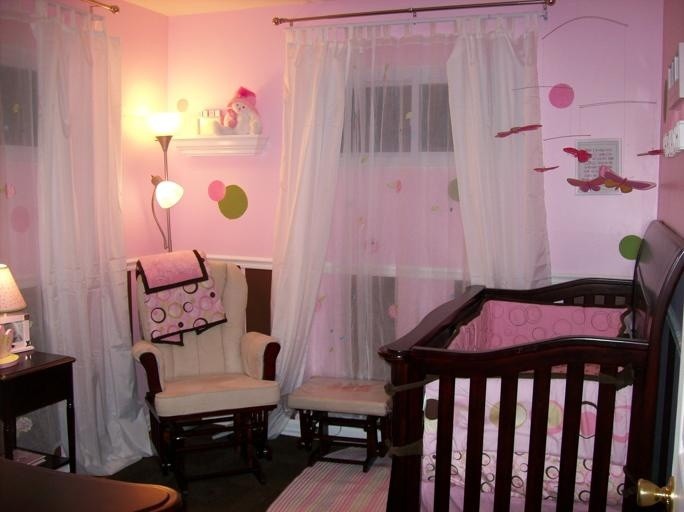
378,221,684,512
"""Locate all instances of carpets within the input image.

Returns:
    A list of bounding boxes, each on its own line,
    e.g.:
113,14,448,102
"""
267,447,394,512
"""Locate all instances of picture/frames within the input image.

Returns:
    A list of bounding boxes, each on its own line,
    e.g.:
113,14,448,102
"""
0,316,34,353
575,140,622,197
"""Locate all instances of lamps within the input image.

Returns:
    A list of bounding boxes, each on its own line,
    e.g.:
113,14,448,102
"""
0,264,27,369
150,109,184,252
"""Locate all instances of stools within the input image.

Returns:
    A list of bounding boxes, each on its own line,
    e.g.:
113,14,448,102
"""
288,379,394,473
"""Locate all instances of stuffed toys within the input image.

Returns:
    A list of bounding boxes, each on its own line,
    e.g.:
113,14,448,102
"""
210,85,264,135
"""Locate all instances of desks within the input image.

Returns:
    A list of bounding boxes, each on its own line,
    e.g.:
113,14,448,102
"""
0,457,182,512
0,351,77,473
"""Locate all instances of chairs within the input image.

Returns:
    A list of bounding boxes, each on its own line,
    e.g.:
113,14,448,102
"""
128,261,281,494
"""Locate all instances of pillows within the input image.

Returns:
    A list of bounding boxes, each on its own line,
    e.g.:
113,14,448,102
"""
447,300,630,374
